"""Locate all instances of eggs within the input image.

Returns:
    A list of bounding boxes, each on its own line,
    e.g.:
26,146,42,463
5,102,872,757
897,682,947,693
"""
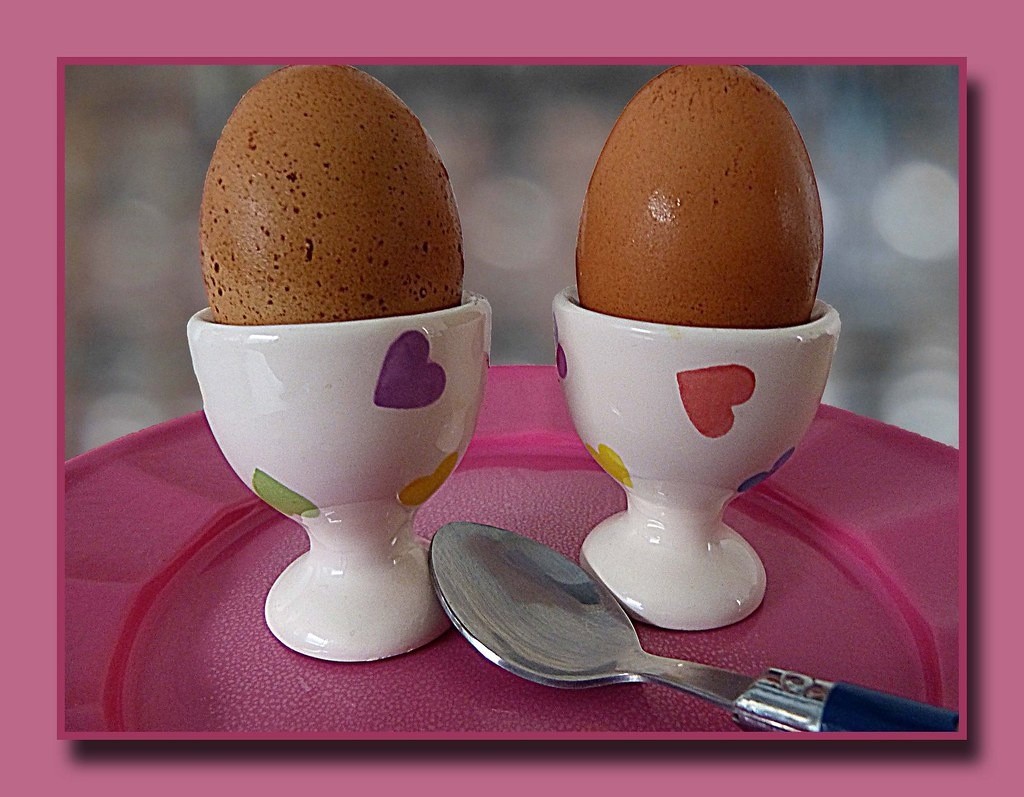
197,63,465,325
572,60,825,326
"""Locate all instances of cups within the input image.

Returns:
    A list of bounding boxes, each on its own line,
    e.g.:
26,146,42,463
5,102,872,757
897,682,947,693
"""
552,287,840,631
186,291,491,663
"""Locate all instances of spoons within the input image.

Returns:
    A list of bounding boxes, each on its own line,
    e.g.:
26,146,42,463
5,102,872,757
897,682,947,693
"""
428,521,959,730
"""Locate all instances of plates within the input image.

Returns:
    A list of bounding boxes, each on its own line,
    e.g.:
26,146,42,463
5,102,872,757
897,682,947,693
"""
65,366,959,730
